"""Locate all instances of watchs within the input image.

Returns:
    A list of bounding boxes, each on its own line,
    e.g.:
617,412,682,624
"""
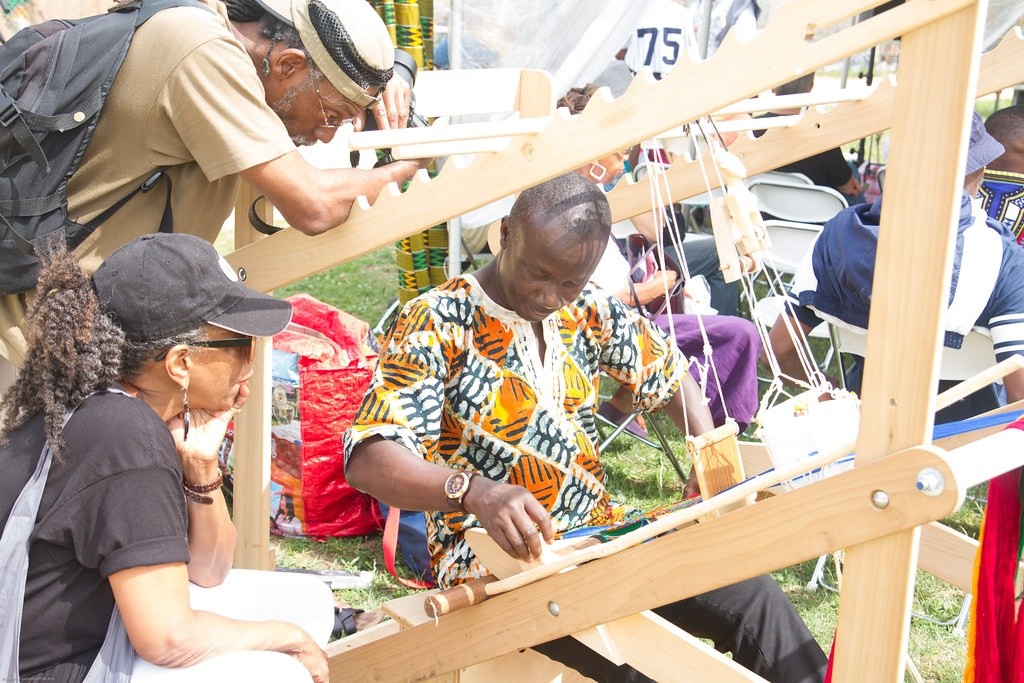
445,471,481,515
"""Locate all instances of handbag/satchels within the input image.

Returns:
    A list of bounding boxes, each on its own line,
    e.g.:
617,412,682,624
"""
220,293,385,541
625,231,686,320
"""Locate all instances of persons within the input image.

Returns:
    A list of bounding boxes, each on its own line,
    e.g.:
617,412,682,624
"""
629,143,740,315
752,72,866,207
762,110,1024,426
556,84,759,436
1,234,335,683
616,0,694,78
345,175,828,683
0,0,434,392
976,106,1024,248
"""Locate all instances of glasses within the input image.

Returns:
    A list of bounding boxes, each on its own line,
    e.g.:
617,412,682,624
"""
307,57,345,129
154,334,254,367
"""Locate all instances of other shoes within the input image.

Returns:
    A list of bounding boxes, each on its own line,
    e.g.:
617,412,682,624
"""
598,402,649,439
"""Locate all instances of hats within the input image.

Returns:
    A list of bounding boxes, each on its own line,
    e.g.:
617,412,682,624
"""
258,0,396,110
964,112,1005,176
87,234,294,343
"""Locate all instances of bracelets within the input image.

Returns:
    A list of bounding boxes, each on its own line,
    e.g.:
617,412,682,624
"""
182,468,223,504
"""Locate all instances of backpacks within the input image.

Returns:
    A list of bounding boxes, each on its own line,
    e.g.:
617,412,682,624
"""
0,0,228,295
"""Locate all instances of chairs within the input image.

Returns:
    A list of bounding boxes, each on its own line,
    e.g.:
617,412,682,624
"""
585,168,1000,629
